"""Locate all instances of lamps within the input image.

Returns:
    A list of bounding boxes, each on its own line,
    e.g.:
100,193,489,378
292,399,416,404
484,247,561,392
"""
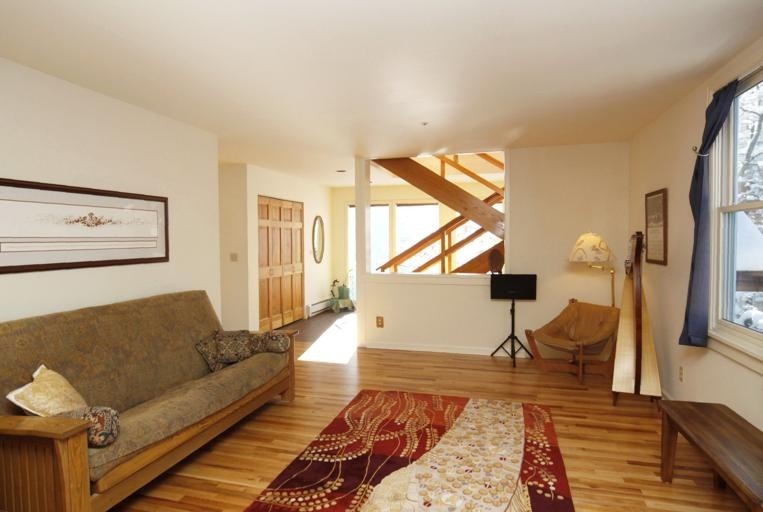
568,232,617,308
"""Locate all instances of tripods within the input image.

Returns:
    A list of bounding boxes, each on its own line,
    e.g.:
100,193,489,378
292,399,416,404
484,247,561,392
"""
491,299,533,367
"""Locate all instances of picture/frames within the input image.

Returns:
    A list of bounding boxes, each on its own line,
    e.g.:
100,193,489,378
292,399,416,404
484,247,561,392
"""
0,179,169,274
645,186,668,266
313,215,325,263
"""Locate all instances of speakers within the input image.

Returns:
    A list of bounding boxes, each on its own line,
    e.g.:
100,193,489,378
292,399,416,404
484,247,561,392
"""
491,274,536,300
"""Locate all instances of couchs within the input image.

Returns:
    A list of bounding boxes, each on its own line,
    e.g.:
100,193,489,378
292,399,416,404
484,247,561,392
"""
1,290,295,512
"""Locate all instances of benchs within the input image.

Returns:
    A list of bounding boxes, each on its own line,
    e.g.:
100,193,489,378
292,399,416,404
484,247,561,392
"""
656,397,763,512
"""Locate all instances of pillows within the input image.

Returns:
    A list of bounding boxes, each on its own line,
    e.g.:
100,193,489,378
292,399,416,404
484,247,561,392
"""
197,327,291,372
5,363,119,447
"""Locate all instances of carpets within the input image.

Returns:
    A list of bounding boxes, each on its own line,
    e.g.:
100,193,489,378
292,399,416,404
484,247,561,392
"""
240,390,576,512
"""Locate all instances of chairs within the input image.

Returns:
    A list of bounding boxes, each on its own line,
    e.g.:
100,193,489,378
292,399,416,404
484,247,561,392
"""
525,299,621,383
